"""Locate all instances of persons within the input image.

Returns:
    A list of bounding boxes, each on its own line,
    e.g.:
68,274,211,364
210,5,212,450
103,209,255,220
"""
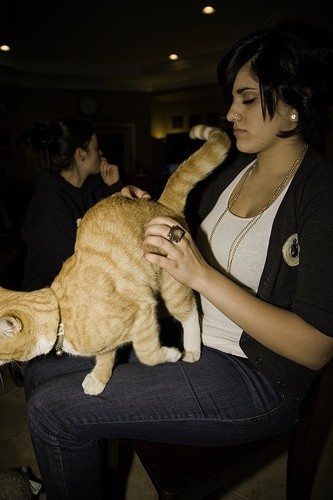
22,120,123,292
23,28,333,500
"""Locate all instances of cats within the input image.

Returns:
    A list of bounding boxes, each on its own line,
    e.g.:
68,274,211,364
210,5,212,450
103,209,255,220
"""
1,124,231,395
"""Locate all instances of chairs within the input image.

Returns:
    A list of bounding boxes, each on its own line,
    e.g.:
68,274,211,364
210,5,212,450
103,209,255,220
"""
124,365,332,500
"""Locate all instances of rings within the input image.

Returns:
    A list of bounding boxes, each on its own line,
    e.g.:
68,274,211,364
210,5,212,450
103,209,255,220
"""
168,225,185,243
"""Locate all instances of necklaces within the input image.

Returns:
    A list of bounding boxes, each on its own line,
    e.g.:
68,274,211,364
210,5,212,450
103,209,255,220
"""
209,143,308,277
